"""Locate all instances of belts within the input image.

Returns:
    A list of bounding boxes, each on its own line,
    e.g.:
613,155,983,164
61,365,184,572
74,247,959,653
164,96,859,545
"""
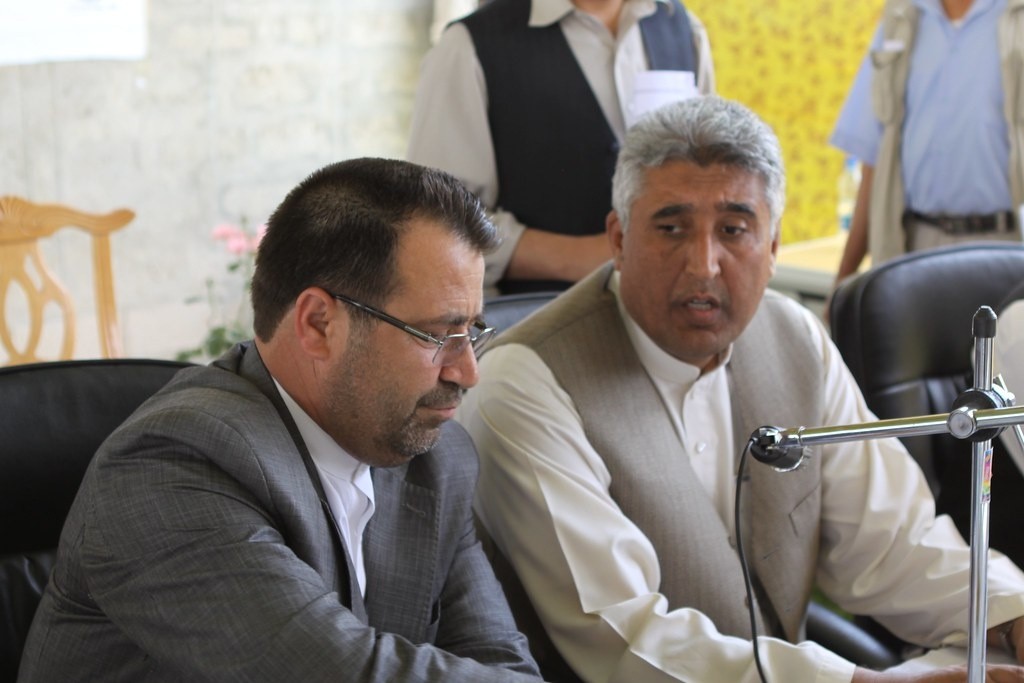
904,209,1015,236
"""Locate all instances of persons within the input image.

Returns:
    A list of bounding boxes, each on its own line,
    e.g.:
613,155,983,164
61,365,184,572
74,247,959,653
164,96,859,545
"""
824,0,1024,323
453,94,1024,683
404,0,714,297
17,158,542,683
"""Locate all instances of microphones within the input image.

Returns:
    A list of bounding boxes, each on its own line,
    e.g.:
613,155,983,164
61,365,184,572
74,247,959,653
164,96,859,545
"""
750,426,812,475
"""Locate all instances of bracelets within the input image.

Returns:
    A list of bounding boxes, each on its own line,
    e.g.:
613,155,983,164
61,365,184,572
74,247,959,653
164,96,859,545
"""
998,622,1016,657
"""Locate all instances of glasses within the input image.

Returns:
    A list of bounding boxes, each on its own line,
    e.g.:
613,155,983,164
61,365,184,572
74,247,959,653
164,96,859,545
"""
332,294,497,366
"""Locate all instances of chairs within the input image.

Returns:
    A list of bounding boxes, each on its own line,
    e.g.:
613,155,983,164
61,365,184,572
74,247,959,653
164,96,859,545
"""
0,195,1024,683
